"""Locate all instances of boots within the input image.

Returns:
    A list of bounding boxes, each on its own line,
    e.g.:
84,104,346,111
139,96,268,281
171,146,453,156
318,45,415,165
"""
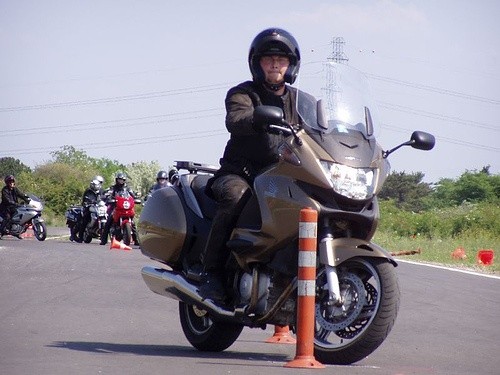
200,208,236,309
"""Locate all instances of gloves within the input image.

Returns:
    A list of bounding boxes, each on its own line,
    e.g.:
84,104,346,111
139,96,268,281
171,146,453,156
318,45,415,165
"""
13,203,19,208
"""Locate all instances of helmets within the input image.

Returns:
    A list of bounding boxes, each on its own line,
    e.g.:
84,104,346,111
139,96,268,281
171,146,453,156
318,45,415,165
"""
93,176,103,182
90,180,100,190
169,170,178,180
5,175,15,184
116,173,125,183
249,28,301,85
157,170,167,181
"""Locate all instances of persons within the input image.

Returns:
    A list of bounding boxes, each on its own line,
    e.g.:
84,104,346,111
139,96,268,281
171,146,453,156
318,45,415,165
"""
154,171,173,190
72,172,139,246
198,28,318,298
0,174,31,239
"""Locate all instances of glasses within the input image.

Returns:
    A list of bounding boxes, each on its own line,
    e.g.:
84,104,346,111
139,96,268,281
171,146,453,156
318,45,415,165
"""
259,56,290,65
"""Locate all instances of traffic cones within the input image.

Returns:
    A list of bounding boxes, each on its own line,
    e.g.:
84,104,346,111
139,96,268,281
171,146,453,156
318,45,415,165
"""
477,250,494,265
110,235,132,250
452,247,467,261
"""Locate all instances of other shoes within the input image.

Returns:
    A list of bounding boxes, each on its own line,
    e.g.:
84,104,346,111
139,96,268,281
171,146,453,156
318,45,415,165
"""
16,234,24,239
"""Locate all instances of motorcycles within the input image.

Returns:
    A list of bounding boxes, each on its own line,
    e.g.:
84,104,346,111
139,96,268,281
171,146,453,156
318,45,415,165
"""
0,193,47,241
66,191,142,246
137,60,436,365
